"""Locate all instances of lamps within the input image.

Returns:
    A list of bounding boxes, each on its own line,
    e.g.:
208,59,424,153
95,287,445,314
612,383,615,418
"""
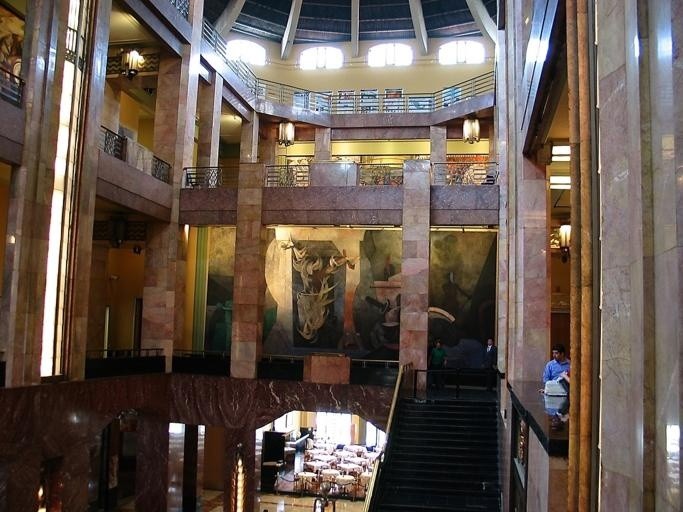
558,224,572,264
120,49,145,79
279,122,296,147
462,116,481,143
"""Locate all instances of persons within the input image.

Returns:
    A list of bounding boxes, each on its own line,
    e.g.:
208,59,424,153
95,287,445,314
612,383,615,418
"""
427,340,448,393
542,343,571,393
482,337,497,392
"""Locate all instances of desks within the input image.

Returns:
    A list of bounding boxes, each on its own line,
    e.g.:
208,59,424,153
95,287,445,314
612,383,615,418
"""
294,439,376,500
